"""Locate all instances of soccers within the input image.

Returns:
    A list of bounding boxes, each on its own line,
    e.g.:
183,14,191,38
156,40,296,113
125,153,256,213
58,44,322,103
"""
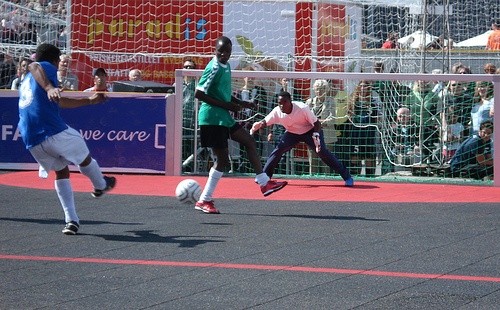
175,179,202,205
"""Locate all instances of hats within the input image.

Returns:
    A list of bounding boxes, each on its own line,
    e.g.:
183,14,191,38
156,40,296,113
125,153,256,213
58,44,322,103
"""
92,67,107,77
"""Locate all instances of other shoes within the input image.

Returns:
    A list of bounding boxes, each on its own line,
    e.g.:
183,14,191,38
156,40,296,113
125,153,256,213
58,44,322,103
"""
344,178,353,187
255,178,258,183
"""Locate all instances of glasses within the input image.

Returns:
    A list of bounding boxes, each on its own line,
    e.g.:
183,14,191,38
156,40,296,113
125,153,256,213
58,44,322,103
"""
183,64,193,69
249,90,252,100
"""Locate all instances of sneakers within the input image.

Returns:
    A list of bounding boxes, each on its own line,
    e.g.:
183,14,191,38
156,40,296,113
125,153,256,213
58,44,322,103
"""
261,180,288,196
91,175,115,197
195,199,220,214
61,221,79,235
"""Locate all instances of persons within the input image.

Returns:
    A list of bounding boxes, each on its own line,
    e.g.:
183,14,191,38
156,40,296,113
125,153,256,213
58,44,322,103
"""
18,44,116,234
349,81,377,177
305,80,338,176
252,92,354,187
83,67,112,91
485,18,500,50
174,59,202,173
194,36,288,213
268,78,303,174
445,38,455,50
372,63,496,182
382,32,400,48
0,0,67,47
129,69,141,81
56,54,78,91
234,66,268,172
11,58,32,90
